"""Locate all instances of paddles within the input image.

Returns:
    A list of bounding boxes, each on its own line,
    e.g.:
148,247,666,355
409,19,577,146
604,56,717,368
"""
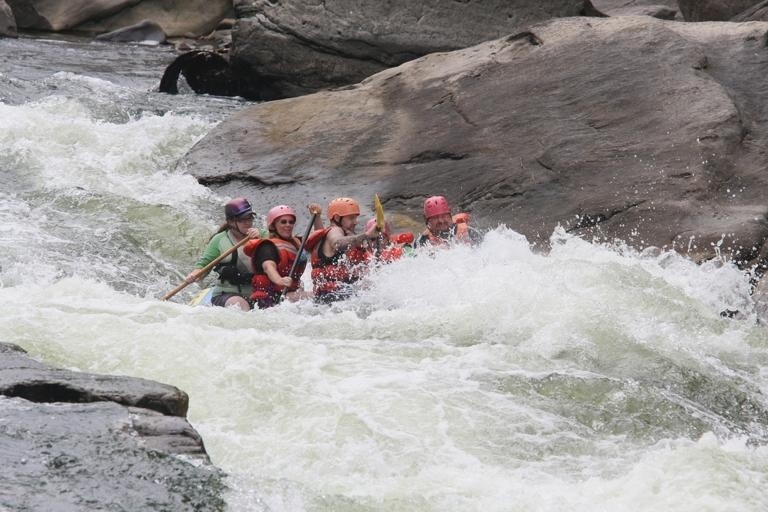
375,194,385,252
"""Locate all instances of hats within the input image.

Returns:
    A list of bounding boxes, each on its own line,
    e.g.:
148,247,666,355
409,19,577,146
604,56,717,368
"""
238,209,256,219
367,218,389,236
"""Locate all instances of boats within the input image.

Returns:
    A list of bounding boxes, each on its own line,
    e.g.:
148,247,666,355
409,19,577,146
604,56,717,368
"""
189,281,217,309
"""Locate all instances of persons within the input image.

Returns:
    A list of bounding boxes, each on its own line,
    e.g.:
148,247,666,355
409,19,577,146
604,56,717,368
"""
304,197,380,306
414,195,482,248
242,202,325,310
185,197,268,311
360,217,412,264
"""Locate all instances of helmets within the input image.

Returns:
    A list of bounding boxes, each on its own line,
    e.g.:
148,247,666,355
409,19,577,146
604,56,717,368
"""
225,198,251,217
268,206,296,233
328,198,360,218
424,195,450,220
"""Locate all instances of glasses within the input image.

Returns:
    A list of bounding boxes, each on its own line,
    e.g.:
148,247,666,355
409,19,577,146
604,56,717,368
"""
280,220,294,225
